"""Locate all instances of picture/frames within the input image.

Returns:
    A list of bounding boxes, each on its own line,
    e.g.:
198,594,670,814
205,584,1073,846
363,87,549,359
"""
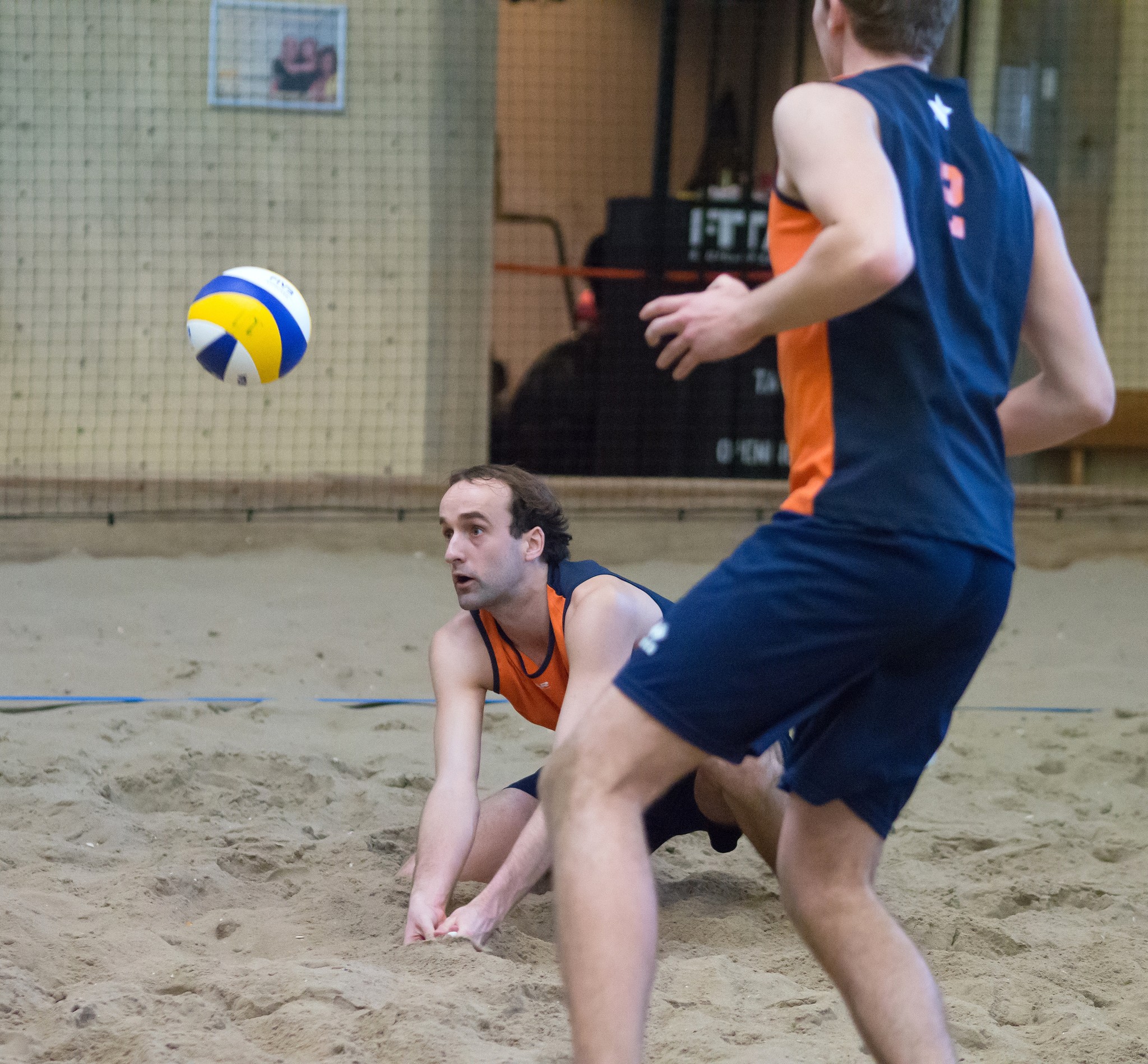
206,0,347,112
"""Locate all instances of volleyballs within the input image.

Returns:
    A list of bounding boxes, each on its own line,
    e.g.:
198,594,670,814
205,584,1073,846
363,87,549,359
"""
187,265,312,386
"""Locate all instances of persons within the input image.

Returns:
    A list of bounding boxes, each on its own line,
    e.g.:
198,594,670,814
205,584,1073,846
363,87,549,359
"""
539,0,1117,1064
264,39,339,105
398,465,789,948
